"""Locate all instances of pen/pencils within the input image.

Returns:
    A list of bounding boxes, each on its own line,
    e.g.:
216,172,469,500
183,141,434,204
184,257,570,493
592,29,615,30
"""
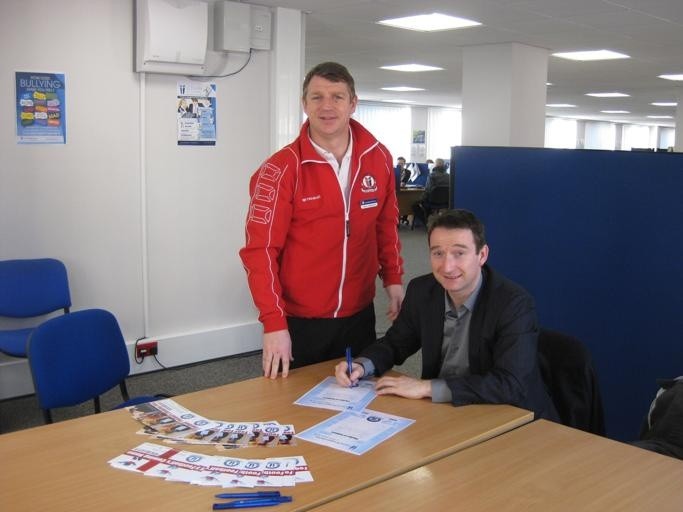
212,491,292,510
345,346,353,389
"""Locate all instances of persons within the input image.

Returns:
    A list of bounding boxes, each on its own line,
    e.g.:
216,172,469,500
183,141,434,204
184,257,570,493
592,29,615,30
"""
331,209,560,424
235,62,405,380
412,159,449,230
395,158,410,188
425,160,434,163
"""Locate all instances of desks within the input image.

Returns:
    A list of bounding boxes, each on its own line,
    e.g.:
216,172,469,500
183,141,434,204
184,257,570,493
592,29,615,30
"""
300,418,683,512
395,188,425,226
0,354,535,512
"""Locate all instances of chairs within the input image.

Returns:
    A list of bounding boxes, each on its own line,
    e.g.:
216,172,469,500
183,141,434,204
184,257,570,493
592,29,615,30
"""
411,185,450,231
0,258,72,359
26,308,177,425
537,328,605,437
